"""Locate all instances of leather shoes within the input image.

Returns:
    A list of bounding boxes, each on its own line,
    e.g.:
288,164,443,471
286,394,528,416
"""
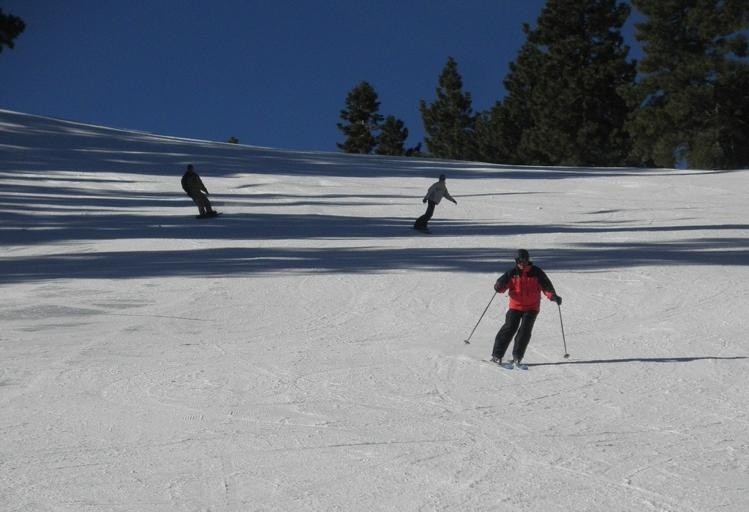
550,295,562,305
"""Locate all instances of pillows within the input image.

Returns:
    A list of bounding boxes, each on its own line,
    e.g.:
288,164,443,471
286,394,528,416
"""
514,249,529,264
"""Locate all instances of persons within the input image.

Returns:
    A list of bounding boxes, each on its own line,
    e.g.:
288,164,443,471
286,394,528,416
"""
415,174,457,228
181,164,212,215
492,249,561,364
